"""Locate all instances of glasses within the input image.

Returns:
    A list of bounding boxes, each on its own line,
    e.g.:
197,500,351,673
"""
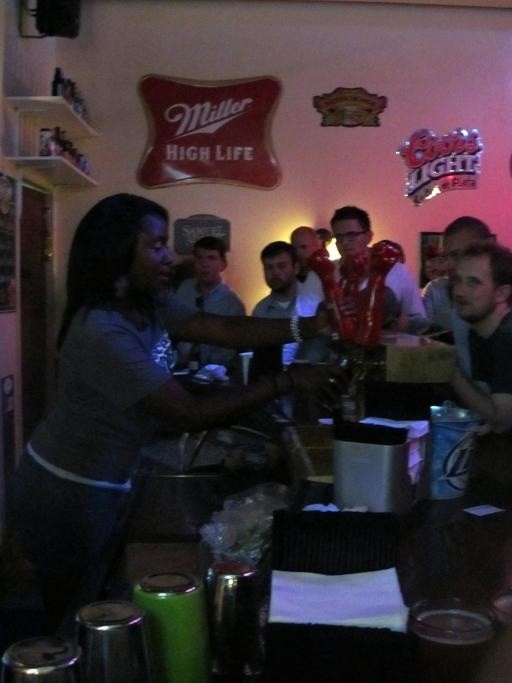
331,229,367,241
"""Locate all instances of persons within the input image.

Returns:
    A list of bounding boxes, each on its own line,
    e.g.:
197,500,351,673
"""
14,193,511,636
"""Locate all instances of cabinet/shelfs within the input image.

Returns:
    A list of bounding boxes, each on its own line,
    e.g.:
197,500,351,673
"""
4,95,100,192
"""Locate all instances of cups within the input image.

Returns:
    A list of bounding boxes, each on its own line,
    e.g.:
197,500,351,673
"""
1,559,264,683
408,586,512,683
